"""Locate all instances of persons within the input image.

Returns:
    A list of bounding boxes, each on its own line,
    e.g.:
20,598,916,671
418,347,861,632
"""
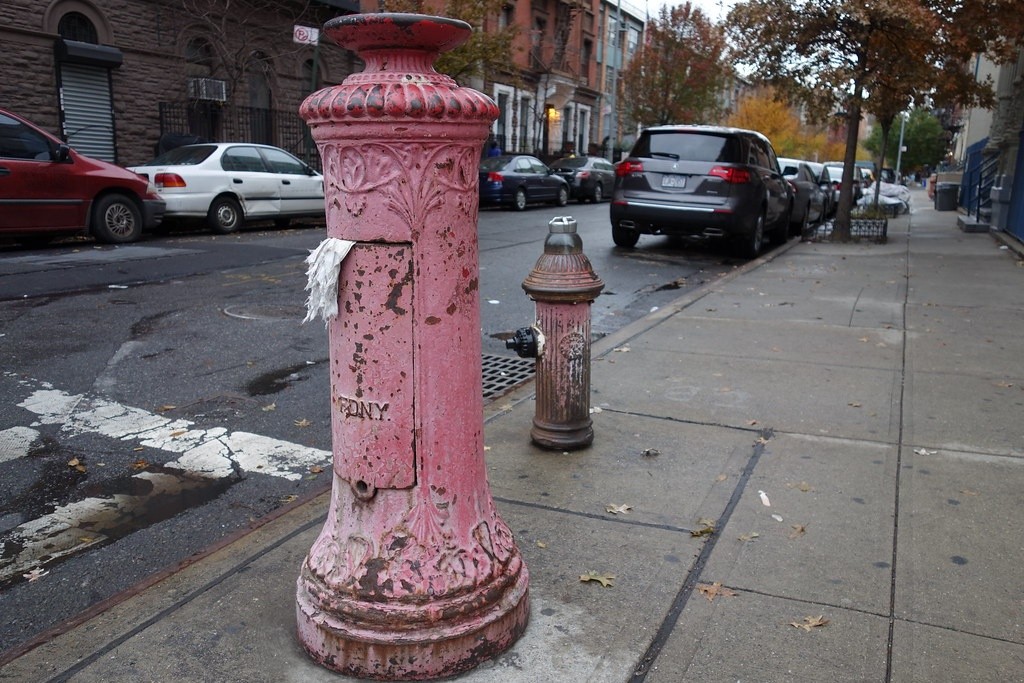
600,136,609,158
490,142,501,158
915,170,921,182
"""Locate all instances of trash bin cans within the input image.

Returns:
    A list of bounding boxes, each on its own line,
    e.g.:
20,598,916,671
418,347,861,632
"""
935,182,962,210
922,178,926,187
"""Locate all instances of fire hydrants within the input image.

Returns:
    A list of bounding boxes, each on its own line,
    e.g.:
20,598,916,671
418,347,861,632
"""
505,216,606,447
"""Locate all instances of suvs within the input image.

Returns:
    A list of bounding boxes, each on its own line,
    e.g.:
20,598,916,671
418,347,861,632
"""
610,124,799,259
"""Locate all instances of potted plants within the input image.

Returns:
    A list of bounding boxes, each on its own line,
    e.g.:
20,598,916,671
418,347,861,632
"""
850,211,885,237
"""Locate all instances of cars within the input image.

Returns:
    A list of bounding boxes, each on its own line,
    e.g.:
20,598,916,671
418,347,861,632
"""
125,142,325,233
776,157,903,236
479,155,571,211
548,155,617,203
0,108,167,244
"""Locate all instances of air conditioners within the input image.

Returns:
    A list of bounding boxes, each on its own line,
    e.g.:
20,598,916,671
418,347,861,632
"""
188,78,226,102
